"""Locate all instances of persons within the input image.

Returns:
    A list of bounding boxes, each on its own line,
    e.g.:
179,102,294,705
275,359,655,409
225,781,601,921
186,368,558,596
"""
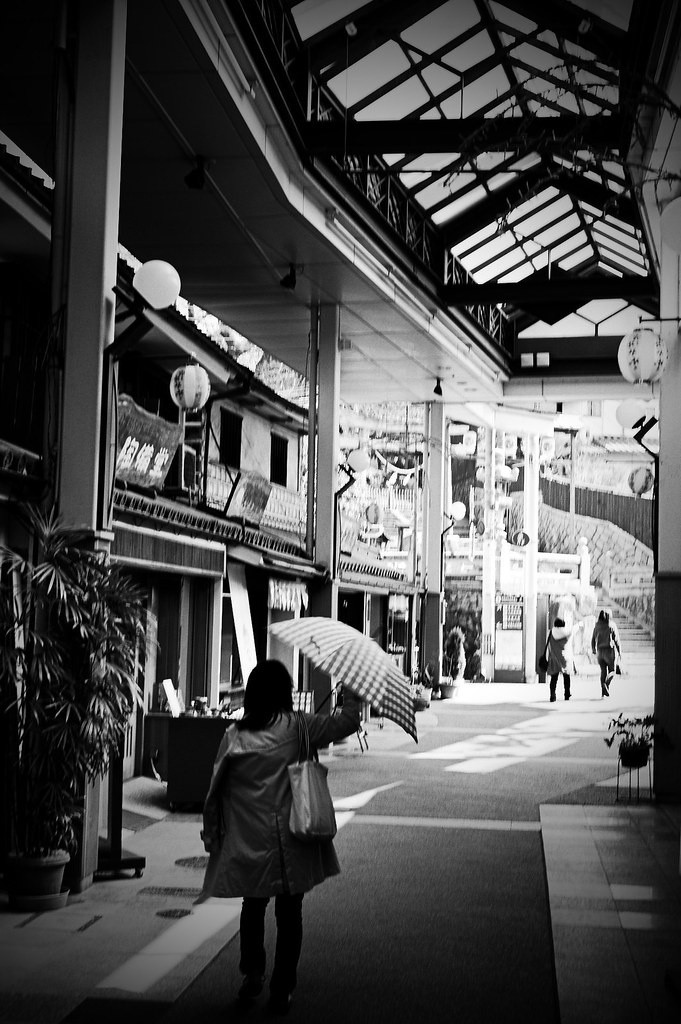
547,617,576,702
591,610,621,698
192,658,362,1007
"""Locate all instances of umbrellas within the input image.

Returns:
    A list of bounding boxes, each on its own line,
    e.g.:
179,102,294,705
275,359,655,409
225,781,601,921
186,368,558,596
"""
268,616,420,744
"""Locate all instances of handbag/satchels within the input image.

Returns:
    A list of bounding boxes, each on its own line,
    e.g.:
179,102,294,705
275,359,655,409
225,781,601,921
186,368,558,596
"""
539,656,548,671
286,712,338,839
616,654,622,674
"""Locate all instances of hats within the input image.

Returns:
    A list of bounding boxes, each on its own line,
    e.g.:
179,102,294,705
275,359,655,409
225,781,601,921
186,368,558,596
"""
554,617,565,627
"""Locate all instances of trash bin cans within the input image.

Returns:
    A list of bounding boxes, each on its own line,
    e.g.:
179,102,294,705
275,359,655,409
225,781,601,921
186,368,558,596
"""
167,714,238,812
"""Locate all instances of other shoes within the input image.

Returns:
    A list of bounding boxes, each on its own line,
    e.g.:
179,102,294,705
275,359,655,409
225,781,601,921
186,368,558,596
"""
600,682,609,697
238,975,266,997
564,693,572,700
262,991,291,1013
550,696,556,702
